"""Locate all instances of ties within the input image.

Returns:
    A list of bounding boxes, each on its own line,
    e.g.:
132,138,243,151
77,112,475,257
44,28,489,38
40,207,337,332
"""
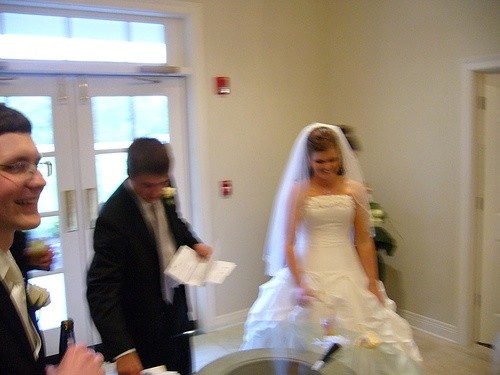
149,203,173,304
6,261,37,352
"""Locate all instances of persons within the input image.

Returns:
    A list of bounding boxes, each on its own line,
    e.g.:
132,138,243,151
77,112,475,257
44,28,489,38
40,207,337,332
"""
86,138,214,375
0,103,105,375
240,123,425,375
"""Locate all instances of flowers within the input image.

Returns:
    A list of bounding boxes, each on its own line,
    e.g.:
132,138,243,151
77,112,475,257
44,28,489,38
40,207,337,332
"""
26,284,51,323
370,202,398,282
162,187,175,205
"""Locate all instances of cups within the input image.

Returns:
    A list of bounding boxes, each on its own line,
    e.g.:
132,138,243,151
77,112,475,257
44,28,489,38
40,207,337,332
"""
30,236,49,267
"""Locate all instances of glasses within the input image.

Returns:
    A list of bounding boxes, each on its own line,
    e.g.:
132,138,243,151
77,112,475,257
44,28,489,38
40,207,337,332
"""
0,156,52,179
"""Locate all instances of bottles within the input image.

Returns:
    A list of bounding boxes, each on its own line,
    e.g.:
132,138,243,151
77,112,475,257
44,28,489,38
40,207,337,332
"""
58,319,75,366
